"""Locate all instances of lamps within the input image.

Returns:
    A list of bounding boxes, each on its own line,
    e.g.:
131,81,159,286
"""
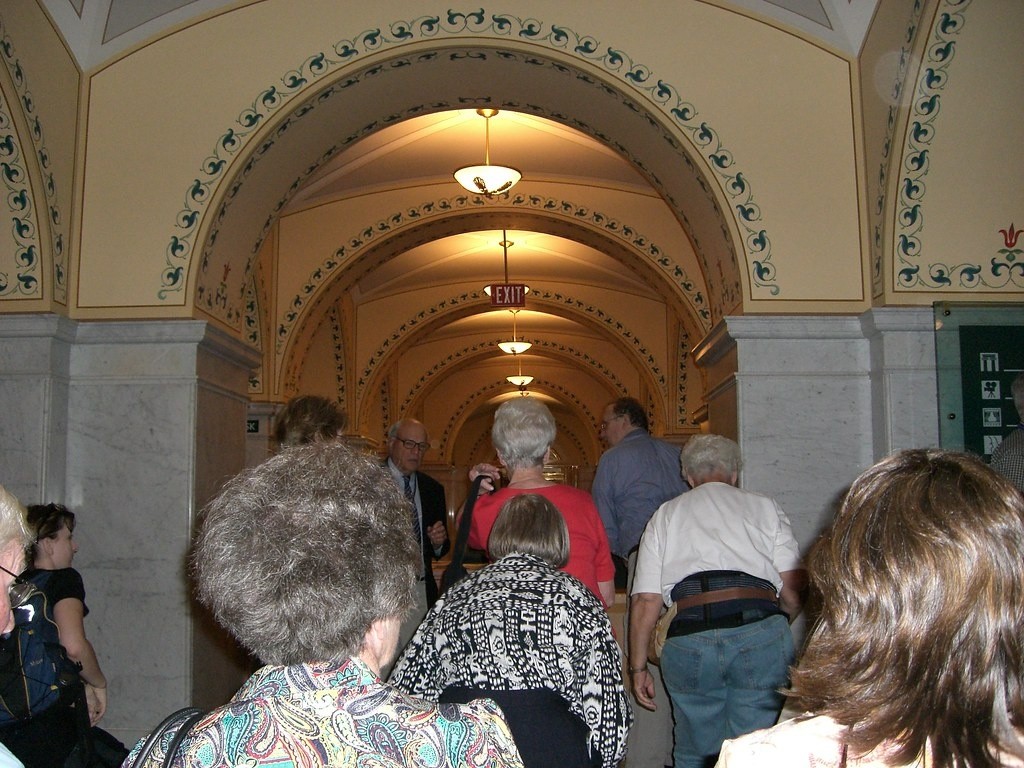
483,241,529,298
497,310,532,356
452,106,523,200
506,356,534,386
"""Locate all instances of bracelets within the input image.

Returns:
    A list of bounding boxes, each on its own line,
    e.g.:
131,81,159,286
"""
630,666,647,673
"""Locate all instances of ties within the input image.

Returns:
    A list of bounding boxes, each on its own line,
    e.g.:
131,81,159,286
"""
402,476,421,555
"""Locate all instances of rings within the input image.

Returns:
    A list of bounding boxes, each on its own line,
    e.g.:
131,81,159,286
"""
96,712,99,714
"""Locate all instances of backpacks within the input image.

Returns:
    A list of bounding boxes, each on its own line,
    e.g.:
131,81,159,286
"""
0,570,86,727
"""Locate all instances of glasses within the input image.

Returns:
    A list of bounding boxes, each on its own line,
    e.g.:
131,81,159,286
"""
600,415,631,431
391,435,430,453
0,566,37,609
36,502,62,543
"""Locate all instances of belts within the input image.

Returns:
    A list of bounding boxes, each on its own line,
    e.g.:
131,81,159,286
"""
627,545,639,559
415,575,424,581
677,588,776,610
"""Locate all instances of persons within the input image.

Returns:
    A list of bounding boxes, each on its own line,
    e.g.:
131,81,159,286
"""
379,418,450,681
630,433,810,768
592,396,692,768
991,374,1024,491
123,441,524,768
389,493,633,768
274,396,348,447
0,485,108,768
457,397,616,638
712,446,1023,768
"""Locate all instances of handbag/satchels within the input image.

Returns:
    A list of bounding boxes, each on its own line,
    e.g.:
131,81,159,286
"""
647,600,678,665
438,475,496,598
